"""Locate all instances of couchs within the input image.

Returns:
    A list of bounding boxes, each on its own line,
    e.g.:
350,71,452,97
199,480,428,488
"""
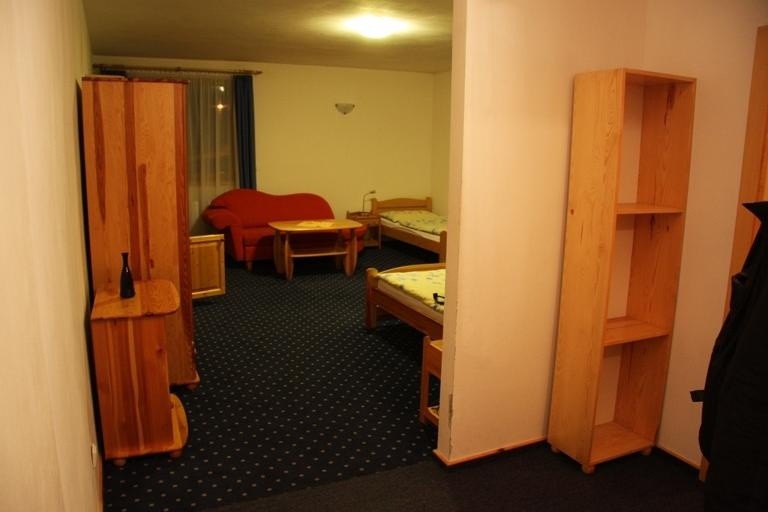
202,188,370,270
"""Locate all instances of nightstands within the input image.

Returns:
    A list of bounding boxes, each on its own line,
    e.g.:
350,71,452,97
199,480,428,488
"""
346,210,383,251
417,334,445,431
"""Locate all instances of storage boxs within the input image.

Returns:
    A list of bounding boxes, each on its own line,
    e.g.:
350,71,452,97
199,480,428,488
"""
189,229,228,303
80,72,199,391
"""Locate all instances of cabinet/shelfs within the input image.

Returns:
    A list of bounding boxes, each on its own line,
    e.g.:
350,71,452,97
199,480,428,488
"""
545,64,700,477
90,276,191,470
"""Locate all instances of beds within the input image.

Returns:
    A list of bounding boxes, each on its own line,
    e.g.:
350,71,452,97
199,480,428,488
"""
365,262,446,340
371,197,447,263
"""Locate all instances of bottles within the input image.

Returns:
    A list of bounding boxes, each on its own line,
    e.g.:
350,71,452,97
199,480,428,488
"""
120,252,136,299
